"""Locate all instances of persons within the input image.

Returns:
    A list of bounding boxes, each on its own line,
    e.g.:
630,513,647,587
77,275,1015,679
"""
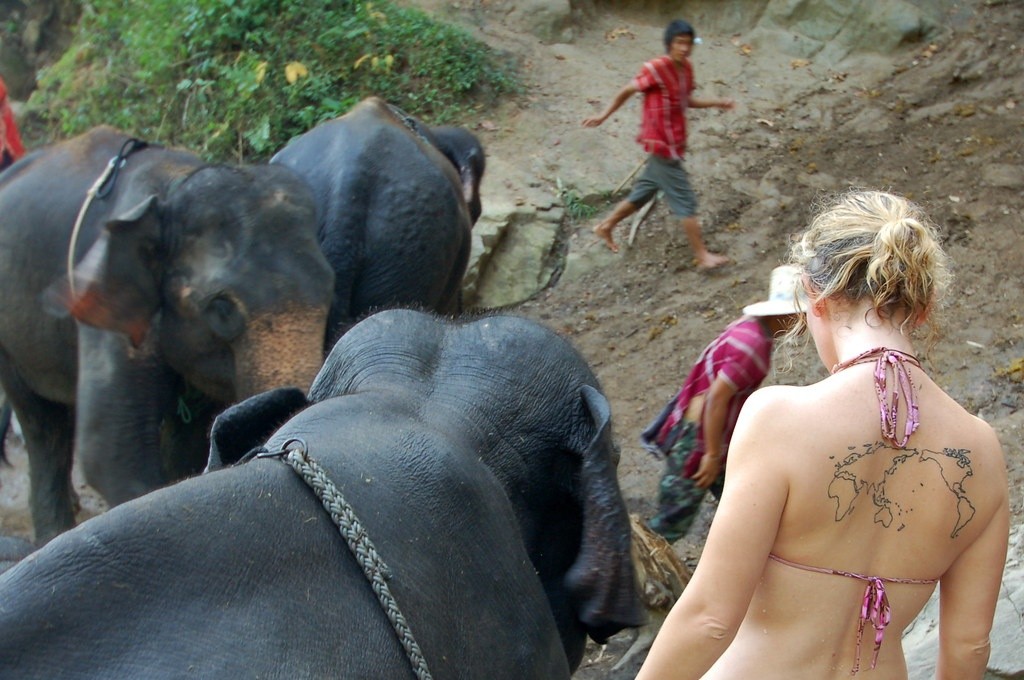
628,190,1009,680
578,19,738,272
643,264,809,545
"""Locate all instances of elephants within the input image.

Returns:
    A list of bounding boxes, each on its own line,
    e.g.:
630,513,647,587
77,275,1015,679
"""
0,95,652,680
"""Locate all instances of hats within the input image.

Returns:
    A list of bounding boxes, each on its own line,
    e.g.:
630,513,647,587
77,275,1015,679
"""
742,263,816,316
